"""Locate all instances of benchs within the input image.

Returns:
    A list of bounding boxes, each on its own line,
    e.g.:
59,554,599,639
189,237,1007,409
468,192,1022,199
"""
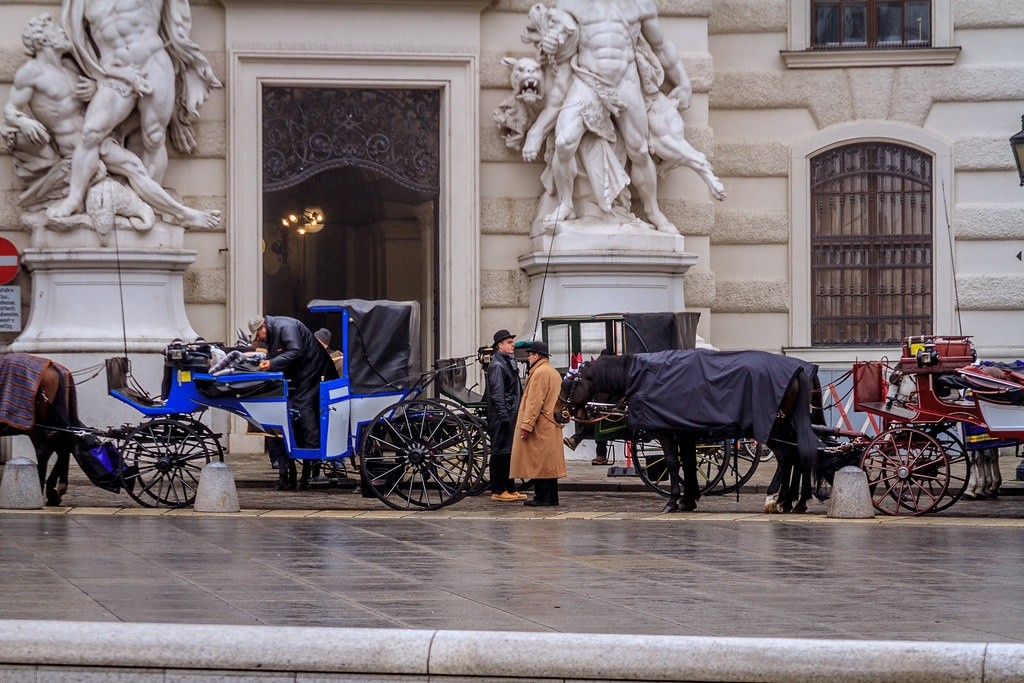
899,335,975,417
162,337,288,403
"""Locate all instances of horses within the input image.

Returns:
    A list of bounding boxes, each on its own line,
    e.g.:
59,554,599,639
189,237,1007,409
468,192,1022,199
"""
552,350,831,512
882,355,1024,501
0,353,79,506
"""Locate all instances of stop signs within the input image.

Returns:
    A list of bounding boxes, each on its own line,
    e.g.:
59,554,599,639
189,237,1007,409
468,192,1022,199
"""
0,236,20,285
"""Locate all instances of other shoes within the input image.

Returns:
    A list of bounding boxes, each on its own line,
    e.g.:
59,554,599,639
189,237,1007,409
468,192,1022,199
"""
491,491,527,501
592,455,608,465
524,496,559,506
564,437,578,451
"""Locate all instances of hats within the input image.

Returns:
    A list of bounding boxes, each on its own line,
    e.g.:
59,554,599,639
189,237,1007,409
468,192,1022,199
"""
248,316,265,341
314,328,332,345
492,330,516,349
525,341,548,356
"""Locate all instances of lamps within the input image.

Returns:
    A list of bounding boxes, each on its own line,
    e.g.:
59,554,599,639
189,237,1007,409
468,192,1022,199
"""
282,206,324,235
916,349,941,367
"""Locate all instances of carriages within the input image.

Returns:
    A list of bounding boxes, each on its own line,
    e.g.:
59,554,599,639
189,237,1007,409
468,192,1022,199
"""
0,299,496,512
552,335,1024,517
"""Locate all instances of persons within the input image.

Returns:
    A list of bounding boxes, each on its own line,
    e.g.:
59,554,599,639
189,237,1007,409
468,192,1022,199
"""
4,0,221,230
563,349,615,465
508,341,568,507
542,0,692,235
248,315,336,470
485,329,528,502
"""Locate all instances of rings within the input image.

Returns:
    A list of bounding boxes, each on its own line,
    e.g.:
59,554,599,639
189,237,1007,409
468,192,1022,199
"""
520,436,524,439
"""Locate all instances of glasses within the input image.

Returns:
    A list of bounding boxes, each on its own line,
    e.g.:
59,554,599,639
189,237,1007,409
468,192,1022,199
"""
528,353,535,356
